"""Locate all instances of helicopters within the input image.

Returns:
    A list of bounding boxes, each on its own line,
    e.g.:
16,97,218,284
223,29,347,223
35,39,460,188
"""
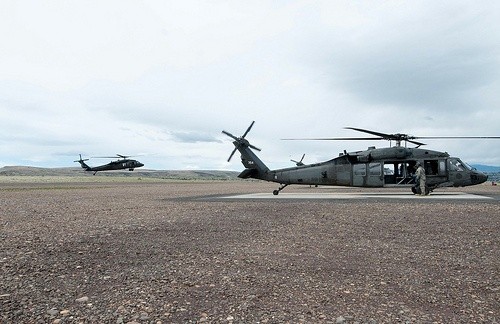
221,120,489,195
75,153,144,176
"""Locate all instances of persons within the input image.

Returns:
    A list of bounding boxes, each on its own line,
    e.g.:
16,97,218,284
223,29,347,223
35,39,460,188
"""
415,162,427,197
